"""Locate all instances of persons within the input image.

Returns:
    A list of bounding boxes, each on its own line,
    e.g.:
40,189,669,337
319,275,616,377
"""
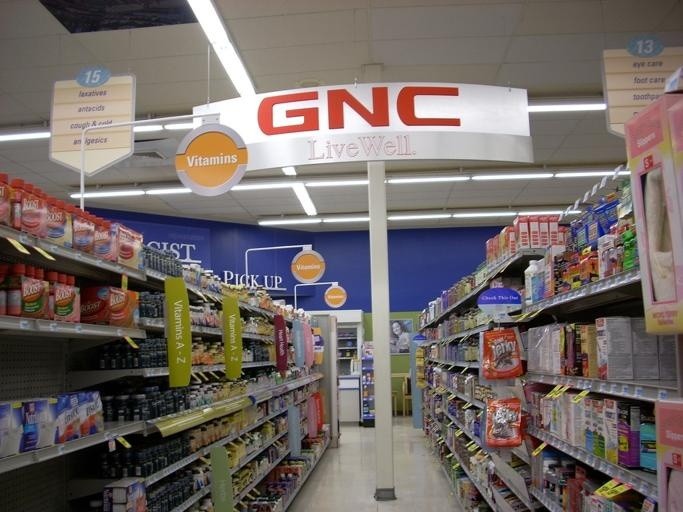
391,321,413,353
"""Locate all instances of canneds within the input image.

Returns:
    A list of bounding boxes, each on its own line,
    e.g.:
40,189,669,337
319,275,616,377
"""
102,386,185,422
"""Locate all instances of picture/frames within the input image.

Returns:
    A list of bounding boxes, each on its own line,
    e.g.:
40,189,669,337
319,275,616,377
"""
390,318,414,356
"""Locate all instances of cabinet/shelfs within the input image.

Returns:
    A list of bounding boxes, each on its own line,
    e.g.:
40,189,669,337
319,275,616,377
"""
254,286,331,512
1,220,147,512
417,248,683,512
65,244,253,512
303,310,374,427
